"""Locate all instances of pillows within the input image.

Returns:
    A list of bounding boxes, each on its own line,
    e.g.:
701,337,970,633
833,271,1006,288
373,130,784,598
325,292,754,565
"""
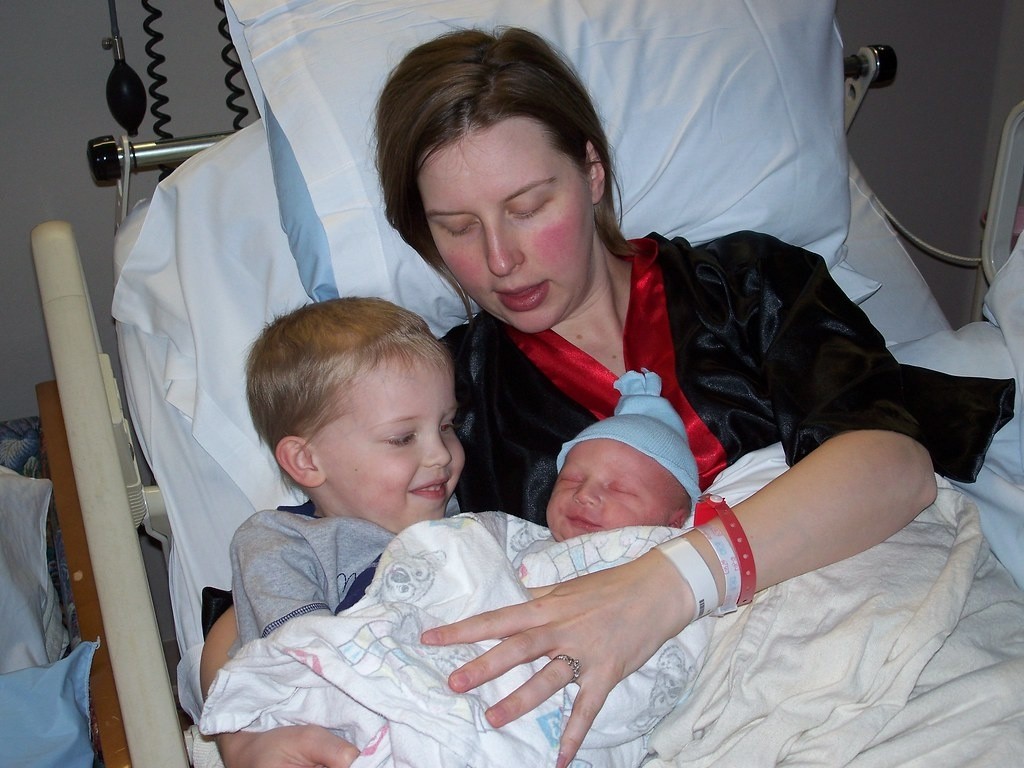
226,1,878,340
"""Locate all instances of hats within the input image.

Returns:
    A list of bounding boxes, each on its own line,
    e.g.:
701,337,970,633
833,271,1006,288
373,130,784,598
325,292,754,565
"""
555,367,702,508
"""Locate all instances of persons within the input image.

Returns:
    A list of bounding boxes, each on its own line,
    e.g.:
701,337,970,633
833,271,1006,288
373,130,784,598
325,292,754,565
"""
509,367,701,593
200,23,1020,768
222,289,465,659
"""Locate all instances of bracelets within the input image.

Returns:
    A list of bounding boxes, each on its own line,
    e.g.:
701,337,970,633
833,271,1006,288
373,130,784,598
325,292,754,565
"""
651,492,756,623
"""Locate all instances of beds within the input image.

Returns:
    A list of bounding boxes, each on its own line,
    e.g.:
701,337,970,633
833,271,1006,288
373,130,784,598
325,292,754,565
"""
28,46,1024,768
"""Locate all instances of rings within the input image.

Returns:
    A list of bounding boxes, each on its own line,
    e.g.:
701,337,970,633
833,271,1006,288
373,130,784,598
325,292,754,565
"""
555,654,583,683
